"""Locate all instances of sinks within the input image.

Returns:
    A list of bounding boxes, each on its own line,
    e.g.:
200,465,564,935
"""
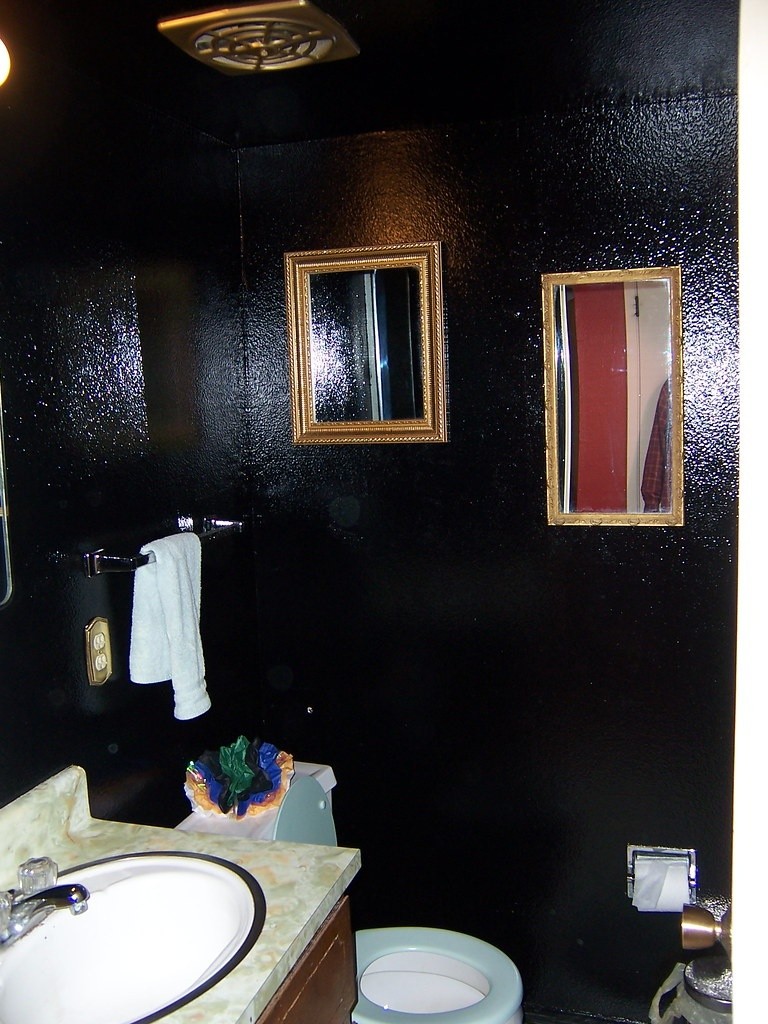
0,845,267,1024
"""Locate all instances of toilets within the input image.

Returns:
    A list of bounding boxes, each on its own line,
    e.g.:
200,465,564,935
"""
175,734,526,1024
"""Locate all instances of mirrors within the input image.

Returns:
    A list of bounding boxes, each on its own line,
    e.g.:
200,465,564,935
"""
283,241,453,444
0,389,13,610
538,265,689,527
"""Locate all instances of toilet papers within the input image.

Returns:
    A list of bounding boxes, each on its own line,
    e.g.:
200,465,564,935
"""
632,856,692,914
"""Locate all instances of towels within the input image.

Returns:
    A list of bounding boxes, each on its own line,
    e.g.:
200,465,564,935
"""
129,525,214,722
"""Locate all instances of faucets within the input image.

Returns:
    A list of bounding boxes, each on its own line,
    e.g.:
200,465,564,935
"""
0,878,91,942
